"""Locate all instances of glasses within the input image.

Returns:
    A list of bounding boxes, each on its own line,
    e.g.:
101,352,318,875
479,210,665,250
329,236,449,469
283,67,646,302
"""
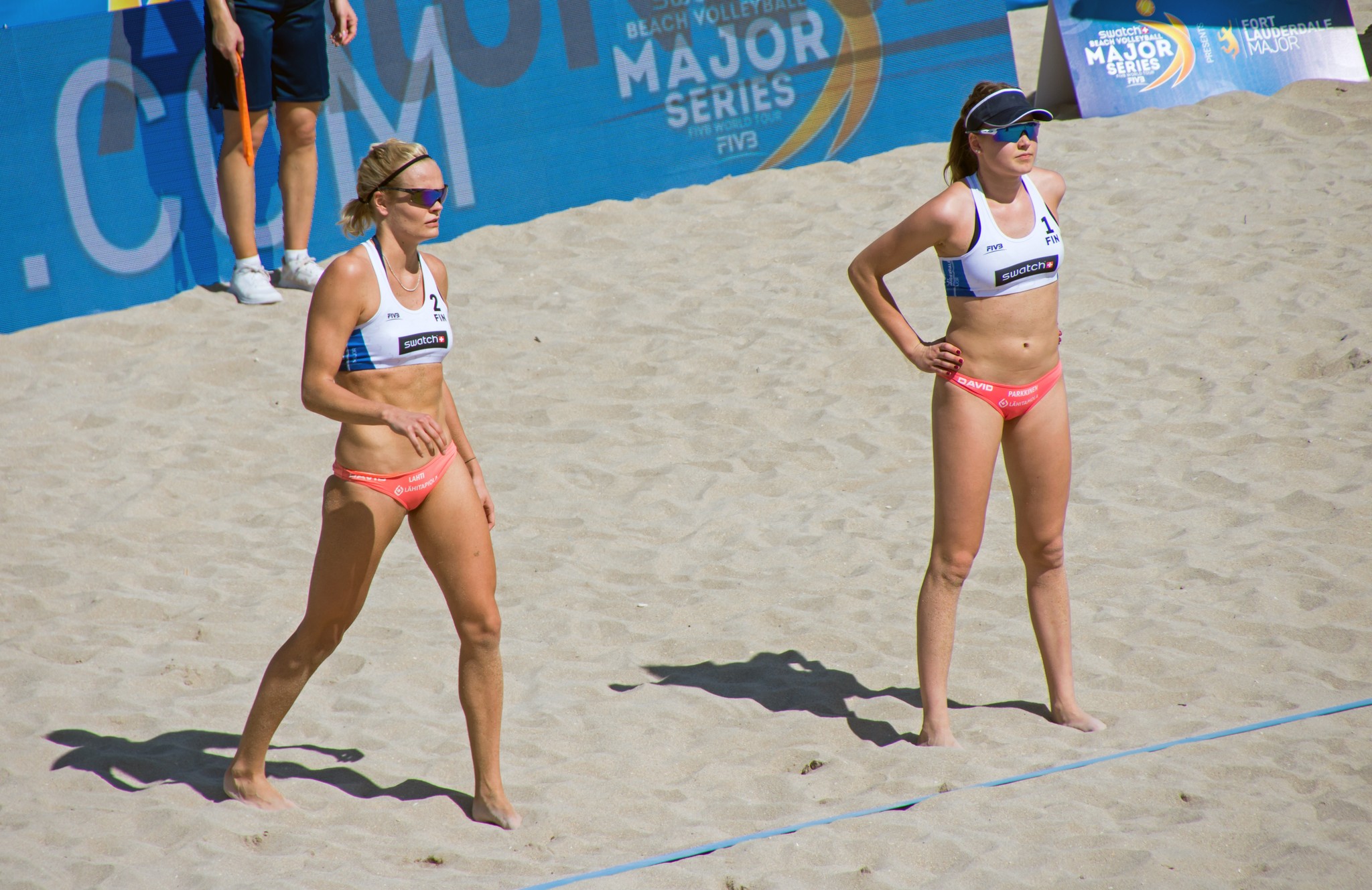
964,120,1041,143
384,184,448,207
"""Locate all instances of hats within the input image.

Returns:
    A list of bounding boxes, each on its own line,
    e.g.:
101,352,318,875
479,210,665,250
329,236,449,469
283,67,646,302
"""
965,88,1053,127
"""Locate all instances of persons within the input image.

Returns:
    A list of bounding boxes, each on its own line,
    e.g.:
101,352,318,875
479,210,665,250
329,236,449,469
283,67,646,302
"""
848,82,1114,752
205,0,359,305
219,139,525,838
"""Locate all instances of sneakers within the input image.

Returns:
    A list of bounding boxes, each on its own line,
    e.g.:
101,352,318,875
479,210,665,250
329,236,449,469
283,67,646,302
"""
229,265,283,304
277,255,324,293
